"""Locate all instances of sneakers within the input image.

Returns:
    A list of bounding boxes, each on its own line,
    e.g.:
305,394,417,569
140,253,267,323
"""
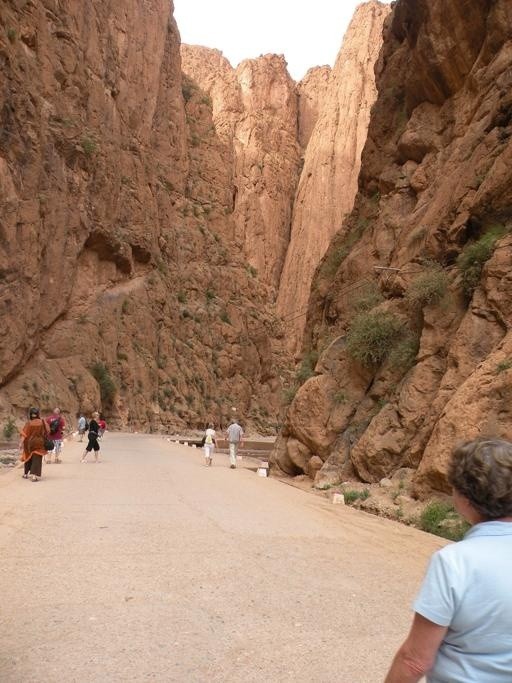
23,473,38,482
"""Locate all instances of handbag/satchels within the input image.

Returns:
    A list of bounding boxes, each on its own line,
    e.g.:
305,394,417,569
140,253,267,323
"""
44,440,54,450
84,423,89,431
205,435,212,445
49,420,59,435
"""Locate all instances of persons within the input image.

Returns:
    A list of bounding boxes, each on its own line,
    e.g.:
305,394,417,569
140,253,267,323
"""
201,421,218,466
18,406,51,482
225,418,244,469
45,408,65,464
382,436,512,683
76,413,89,443
80,411,103,463
97,417,106,442
